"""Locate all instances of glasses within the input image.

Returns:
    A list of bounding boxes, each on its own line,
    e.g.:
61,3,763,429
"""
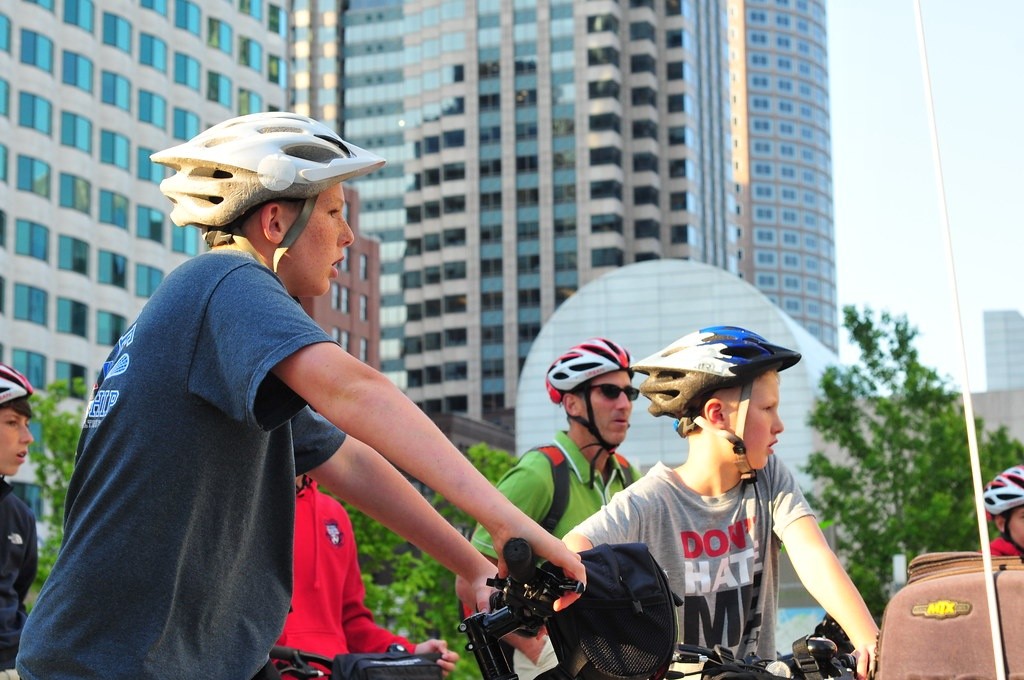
574,383,640,401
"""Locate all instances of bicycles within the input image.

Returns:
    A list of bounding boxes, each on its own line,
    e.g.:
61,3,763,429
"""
675,611,879,680
270,644,443,680
457,537,684,680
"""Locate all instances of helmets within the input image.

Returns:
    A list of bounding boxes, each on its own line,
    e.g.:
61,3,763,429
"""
630,325,802,438
0,362,34,404
983,466,1024,515
149,111,387,228
545,337,631,403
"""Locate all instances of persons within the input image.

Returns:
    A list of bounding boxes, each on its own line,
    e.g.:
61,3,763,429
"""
271,473,460,680
15,110,588,680
978,464,1024,557
454,336,642,680
552,325,882,680
0,360,38,671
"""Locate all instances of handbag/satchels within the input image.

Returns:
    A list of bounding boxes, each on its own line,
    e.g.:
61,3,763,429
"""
540,542,676,680
